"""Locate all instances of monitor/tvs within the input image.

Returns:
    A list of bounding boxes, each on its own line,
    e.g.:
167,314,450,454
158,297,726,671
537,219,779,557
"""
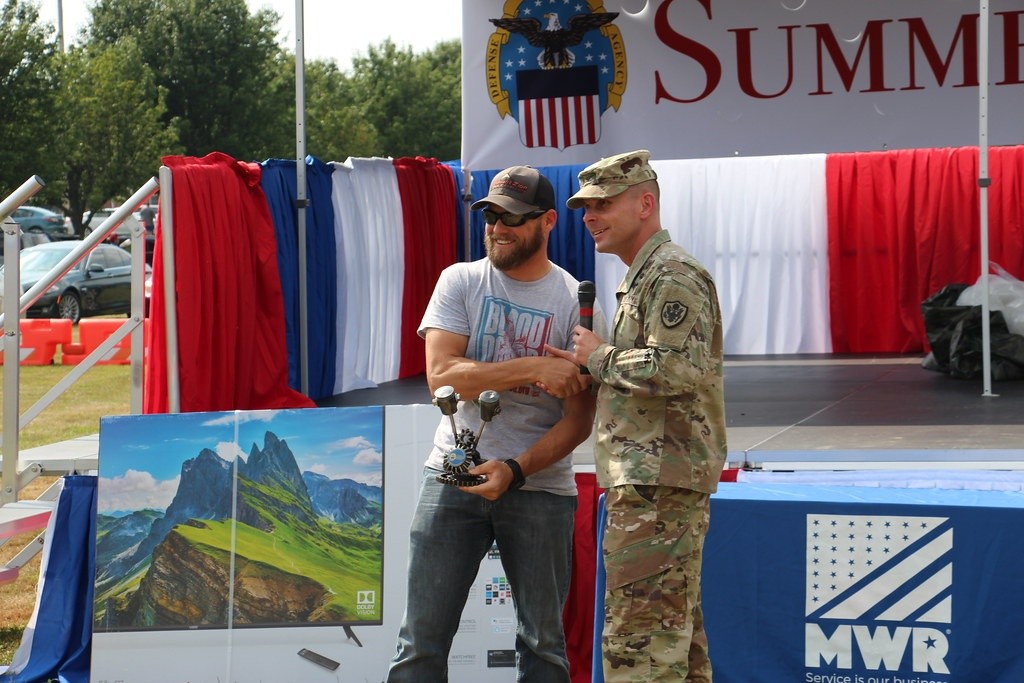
92,404,386,633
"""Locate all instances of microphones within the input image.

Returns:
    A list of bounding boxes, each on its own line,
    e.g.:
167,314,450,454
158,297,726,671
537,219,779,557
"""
577,280,596,375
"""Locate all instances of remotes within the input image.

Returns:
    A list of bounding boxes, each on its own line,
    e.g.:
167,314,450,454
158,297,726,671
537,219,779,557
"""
298,648,341,671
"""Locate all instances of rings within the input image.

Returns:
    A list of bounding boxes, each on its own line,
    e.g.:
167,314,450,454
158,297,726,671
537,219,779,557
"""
573,345,576,349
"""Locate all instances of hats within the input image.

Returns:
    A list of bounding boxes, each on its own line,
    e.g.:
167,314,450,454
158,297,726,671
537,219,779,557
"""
566,150,657,209
471,165,555,215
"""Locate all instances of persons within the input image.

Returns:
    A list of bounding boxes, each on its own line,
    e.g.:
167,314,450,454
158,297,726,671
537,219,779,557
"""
140,203,155,232
537,149,727,683
387,164,608,683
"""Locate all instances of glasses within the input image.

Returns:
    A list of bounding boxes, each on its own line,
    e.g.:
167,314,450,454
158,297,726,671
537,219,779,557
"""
481,206,547,227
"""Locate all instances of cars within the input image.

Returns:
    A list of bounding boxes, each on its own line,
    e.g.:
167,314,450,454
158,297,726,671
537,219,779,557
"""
65,208,132,240
1,240,152,325
12,206,68,238
132,204,159,228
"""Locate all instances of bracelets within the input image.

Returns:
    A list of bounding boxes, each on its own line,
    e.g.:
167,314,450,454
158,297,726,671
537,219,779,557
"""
505,459,525,492
